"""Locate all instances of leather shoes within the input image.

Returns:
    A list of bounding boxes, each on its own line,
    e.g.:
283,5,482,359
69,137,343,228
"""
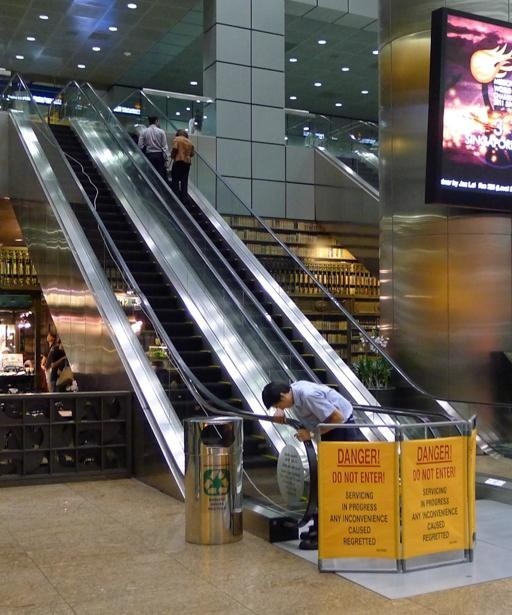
299,540,319,551
299,530,317,540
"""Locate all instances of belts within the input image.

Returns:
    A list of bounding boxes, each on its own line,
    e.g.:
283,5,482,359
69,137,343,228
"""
344,414,355,425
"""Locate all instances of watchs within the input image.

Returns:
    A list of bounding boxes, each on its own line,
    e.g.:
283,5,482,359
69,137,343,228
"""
309,430,317,440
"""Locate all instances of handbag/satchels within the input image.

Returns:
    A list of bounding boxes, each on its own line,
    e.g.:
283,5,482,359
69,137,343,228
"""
56,356,73,387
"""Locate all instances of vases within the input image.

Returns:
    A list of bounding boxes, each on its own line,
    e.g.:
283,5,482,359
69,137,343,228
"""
367,385,398,409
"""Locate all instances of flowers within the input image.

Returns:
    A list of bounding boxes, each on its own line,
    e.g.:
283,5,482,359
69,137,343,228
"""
351,327,392,386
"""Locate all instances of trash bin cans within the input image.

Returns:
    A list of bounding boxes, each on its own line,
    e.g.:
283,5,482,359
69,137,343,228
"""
182,415,245,546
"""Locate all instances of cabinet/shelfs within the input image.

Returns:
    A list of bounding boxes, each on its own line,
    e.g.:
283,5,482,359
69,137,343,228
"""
220,212,379,370
0,245,40,290
1,389,134,485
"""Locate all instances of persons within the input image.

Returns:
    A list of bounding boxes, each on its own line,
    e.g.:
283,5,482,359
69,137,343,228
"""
138,115,171,179
45,339,69,393
261,378,360,551
169,128,196,207
40,331,57,387
5,332,15,354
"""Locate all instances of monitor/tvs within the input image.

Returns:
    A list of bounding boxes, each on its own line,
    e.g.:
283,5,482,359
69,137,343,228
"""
424,8,512,213
